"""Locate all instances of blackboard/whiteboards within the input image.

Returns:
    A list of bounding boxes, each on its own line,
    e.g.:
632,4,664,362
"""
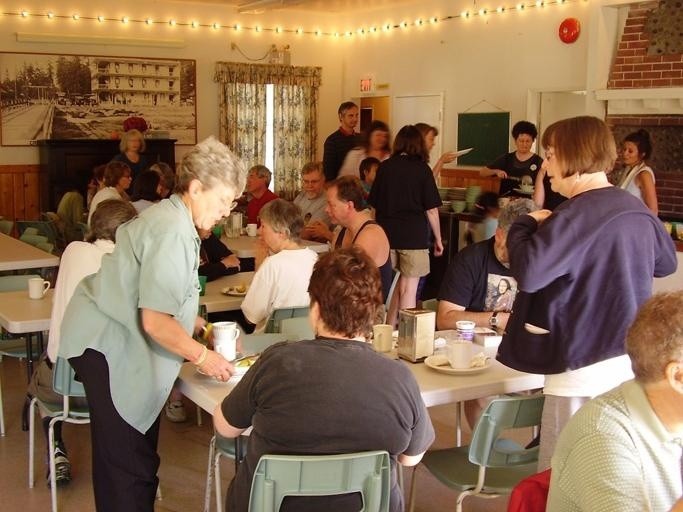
457,111,510,170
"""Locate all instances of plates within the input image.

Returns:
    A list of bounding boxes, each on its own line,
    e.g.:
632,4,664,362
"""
453,148,472,157
448,189,466,200
423,355,493,374
442,199,451,211
438,189,449,199
513,188,535,194
222,286,249,296
393,330,446,348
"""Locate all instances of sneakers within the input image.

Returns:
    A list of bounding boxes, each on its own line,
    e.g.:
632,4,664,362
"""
167,400,188,423
47,447,73,487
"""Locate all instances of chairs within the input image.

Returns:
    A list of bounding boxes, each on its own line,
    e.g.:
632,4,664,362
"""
0,275,49,437
19,234,47,247
203,428,252,512
18,227,40,242
381,263,402,325
26,242,56,280
247,450,391,511
26,335,161,512
263,305,308,333
278,316,315,335
416,299,439,331
0,220,13,236
406,395,545,512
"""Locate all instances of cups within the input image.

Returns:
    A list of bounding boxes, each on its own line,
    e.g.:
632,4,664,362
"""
245,223,258,237
211,322,240,339
498,198,510,208
373,324,394,353
443,340,473,369
230,212,242,239
29,278,50,299
455,321,476,343
213,339,236,361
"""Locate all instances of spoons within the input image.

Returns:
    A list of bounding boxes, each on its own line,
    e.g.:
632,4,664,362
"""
222,286,234,296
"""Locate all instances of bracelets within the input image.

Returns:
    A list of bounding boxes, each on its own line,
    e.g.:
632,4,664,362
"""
190,346,209,367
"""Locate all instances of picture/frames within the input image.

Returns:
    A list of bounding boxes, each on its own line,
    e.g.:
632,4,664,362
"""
0,51,196,148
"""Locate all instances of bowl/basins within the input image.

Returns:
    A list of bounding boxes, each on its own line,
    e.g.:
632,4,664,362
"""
520,185,533,191
465,186,481,210
452,200,466,213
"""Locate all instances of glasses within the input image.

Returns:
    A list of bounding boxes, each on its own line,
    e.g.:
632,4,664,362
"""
207,191,239,213
544,151,556,163
302,179,325,186
123,175,131,179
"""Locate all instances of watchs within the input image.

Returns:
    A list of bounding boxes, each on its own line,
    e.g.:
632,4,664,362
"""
489,305,499,336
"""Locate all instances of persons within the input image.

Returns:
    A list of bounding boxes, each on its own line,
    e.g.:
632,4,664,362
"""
356,158,380,203
545,292,681,508
87,168,129,205
114,131,145,173
323,175,394,310
495,117,677,472
213,249,436,509
366,125,445,332
339,119,391,182
323,103,364,183
240,199,322,337
288,162,332,236
59,135,248,512
480,119,552,212
57,174,84,229
25,199,139,486
615,131,658,217
237,165,280,233
88,162,130,234
437,198,542,328
146,164,172,194
124,171,158,222
413,123,455,187
